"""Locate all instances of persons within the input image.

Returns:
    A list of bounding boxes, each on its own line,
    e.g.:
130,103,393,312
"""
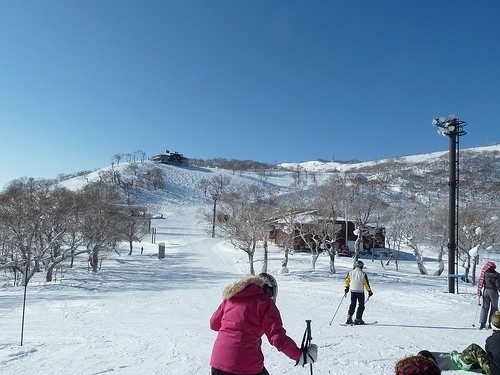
394,262,500,375
210,272,318,375
343,261,373,325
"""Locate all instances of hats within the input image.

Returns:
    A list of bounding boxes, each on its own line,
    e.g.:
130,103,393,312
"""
490,311,500,330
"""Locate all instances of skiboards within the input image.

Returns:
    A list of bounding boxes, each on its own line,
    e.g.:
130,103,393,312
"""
339,321,378,326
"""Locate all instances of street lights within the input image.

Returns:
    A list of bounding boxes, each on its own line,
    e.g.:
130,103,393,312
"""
431,113,466,293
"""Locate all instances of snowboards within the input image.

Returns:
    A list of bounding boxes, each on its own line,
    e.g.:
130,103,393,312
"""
430,352,482,370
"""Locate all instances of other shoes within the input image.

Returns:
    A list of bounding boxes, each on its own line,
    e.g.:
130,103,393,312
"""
355,318,364,324
480,324,485,329
450,350,474,371
347,314,352,324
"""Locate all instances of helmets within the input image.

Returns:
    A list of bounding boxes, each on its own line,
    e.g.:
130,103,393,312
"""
354,261,364,268
259,273,278,298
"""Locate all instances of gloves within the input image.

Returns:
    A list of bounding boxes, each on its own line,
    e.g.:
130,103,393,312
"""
369,291,373,296
345,287,349,293
294,344,318,366
